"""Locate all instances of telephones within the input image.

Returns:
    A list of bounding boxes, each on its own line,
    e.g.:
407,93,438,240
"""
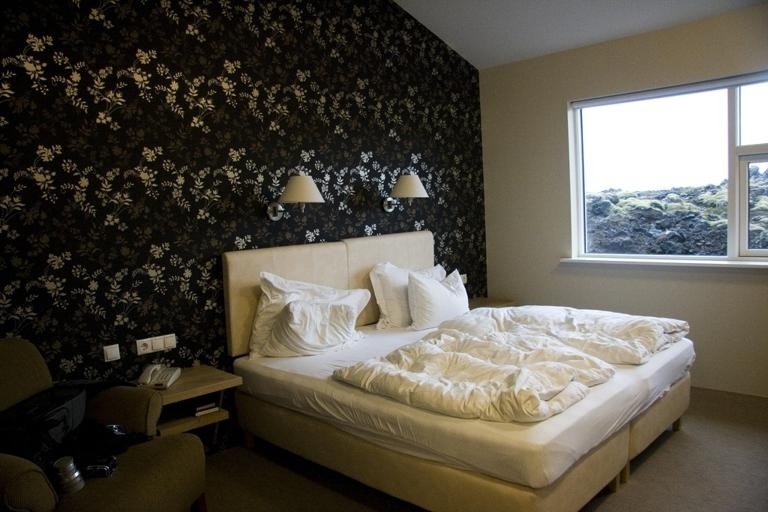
138,364,181,388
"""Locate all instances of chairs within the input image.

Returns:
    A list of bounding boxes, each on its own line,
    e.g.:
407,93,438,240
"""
0,338,209,512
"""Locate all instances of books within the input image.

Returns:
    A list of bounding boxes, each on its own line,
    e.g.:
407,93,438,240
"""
188,402,219,418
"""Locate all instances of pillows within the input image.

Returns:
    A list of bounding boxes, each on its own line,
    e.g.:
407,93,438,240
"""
370,261,447,329
260,300,357,357
249,271,371,360
406,268,469,331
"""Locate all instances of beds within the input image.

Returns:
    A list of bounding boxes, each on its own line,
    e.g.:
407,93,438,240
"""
222,230,696,512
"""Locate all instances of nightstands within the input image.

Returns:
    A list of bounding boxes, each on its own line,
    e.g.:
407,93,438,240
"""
468,296,518,309
130,364,244,446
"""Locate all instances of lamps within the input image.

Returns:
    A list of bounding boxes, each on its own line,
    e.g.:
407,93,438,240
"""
382,174,429,213
266,175,325,222
